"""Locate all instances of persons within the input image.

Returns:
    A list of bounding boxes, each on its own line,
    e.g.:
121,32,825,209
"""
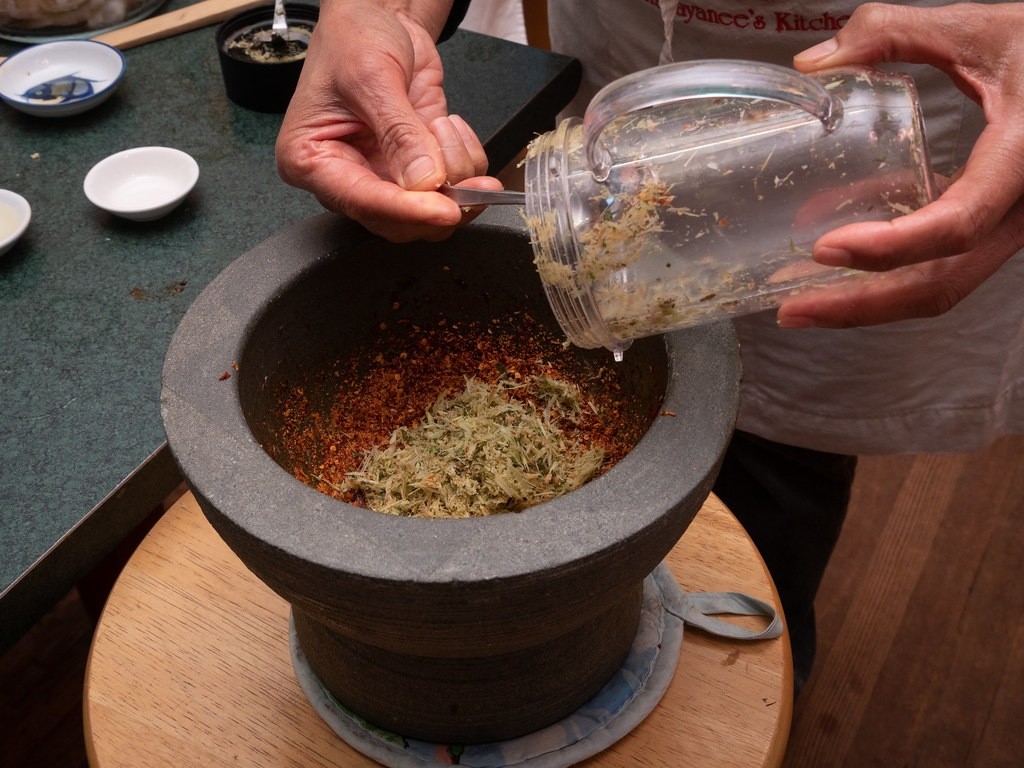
275,0,1024,704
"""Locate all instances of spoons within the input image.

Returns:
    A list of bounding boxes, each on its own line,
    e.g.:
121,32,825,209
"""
438,179,612,243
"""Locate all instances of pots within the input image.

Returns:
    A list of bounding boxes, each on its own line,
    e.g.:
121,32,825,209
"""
0,0,165,43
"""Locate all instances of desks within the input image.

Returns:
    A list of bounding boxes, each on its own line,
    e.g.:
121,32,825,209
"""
0,0,582,598
81,491,792,768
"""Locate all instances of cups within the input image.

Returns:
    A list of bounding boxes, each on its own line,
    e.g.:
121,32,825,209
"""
524,57,935,351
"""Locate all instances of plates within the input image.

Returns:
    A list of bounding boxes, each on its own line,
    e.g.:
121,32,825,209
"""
0,188,32,254
0,41,126,117
84,145,199,221
